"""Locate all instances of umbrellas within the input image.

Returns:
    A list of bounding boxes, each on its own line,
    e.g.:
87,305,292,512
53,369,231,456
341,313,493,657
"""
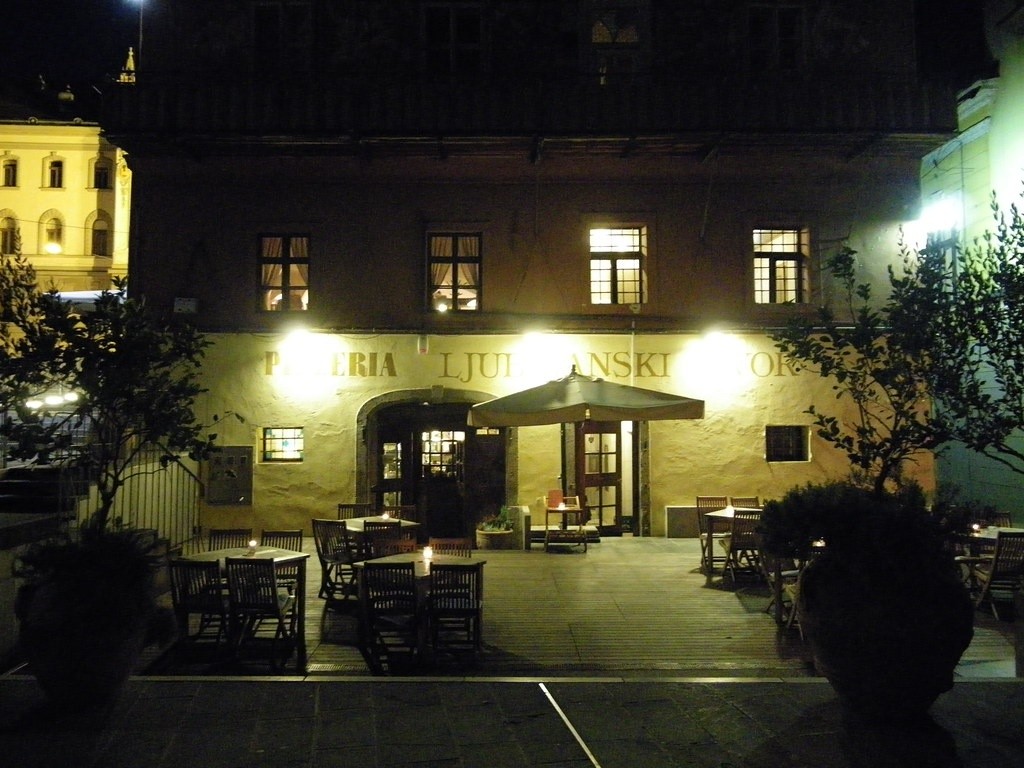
467,365,705,531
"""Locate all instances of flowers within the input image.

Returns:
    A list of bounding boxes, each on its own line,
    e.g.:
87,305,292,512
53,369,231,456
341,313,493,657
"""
475,505,515,532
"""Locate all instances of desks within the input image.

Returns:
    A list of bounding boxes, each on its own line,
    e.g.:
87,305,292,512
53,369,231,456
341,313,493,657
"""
177,545,311,636
352,551,487,652
704,507,760,573
331,513,421,534
959,527,1024,546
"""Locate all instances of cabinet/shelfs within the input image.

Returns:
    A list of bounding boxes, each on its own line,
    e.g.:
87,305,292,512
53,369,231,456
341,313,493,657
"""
543,496,588,552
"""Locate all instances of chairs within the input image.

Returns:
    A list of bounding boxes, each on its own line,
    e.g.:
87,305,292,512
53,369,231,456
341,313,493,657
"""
697,495,825,630
169,499,482,671
954,530,1024,620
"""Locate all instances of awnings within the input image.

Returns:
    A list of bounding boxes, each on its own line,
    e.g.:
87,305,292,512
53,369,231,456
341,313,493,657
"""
41,286,128,314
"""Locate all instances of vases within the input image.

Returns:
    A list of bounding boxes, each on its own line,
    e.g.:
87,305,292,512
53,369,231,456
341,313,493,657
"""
794,507,974,725
476,528,515,550
